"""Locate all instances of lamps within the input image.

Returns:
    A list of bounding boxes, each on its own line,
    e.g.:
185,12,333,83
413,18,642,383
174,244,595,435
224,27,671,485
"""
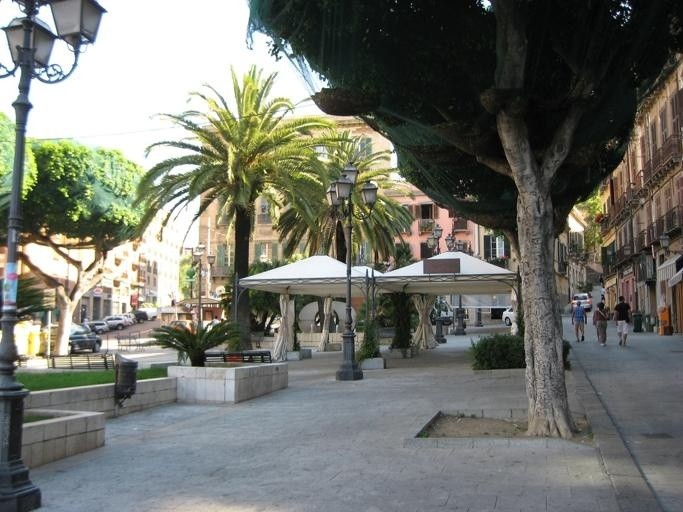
655,234,683,259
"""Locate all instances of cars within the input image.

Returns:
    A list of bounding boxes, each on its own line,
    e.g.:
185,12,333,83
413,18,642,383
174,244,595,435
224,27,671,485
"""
44,307,159,354
503,305,514,326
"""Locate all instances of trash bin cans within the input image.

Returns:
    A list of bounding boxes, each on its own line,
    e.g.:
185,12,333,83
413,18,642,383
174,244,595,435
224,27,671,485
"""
632,312,644,332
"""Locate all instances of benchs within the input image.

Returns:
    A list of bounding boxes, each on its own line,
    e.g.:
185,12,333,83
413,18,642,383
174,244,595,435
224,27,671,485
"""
203,350,273,363
47,354,138,370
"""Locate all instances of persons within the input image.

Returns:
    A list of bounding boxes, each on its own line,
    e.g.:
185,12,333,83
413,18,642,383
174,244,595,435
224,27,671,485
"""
613,295,631,347
570,300,586,342
599,284,606,302
592,301,609,346
570,295,578,312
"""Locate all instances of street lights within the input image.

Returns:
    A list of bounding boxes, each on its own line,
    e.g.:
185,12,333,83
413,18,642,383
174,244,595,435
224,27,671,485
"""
426,220,483,345
191,242,215,330
0,0,106,511
324,158,377,384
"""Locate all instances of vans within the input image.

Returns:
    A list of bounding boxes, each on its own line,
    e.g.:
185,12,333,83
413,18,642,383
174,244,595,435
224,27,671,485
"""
571,292,594,311
